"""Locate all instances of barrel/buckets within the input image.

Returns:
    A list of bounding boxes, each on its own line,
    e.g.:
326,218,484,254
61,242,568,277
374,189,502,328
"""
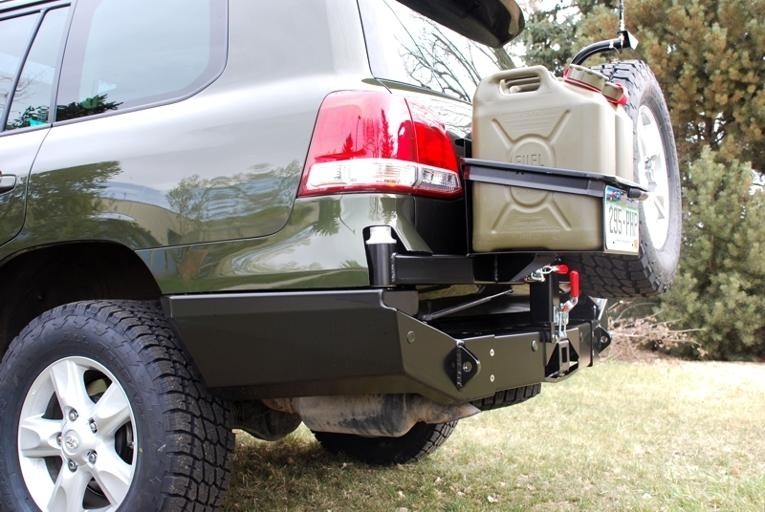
505,73,637,186
464,62,602,254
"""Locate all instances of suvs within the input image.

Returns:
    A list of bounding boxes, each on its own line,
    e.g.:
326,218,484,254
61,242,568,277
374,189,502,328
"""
0,0,684,512
